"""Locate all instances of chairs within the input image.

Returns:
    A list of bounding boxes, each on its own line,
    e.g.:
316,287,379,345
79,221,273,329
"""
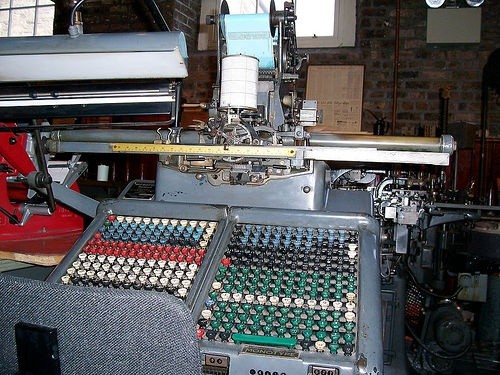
0,273,201,375
